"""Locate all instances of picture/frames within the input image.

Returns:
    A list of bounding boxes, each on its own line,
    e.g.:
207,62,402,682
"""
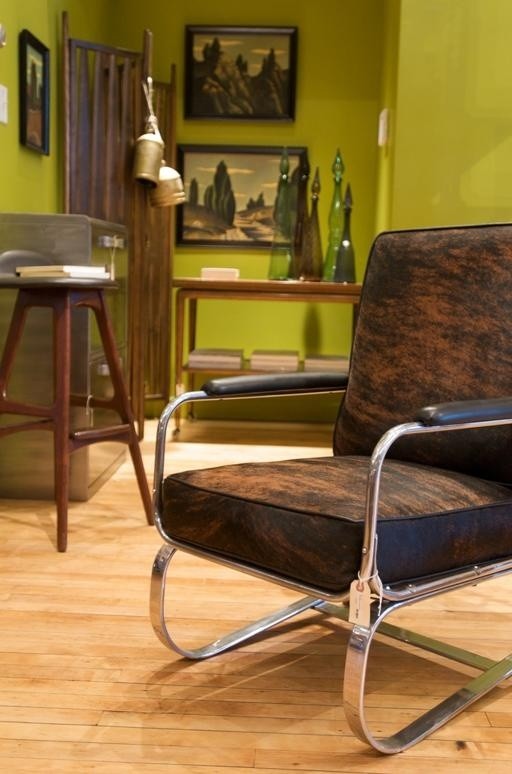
184,25,298,123
176,143,308,247
18,29,50,156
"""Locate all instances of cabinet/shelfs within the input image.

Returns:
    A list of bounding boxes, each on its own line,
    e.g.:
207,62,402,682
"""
0,213,130,501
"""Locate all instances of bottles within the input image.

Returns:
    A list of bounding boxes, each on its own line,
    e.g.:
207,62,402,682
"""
133,127,166,187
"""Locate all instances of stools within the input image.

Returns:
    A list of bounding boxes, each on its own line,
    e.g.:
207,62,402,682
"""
0,250,155,552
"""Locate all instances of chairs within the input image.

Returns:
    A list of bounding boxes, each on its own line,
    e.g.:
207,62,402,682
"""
150,224,512,756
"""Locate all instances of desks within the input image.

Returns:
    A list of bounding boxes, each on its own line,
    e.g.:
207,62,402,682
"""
172,277,363,432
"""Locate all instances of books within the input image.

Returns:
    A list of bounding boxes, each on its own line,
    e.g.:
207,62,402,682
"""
15,263,109,280
187,348,351,375
